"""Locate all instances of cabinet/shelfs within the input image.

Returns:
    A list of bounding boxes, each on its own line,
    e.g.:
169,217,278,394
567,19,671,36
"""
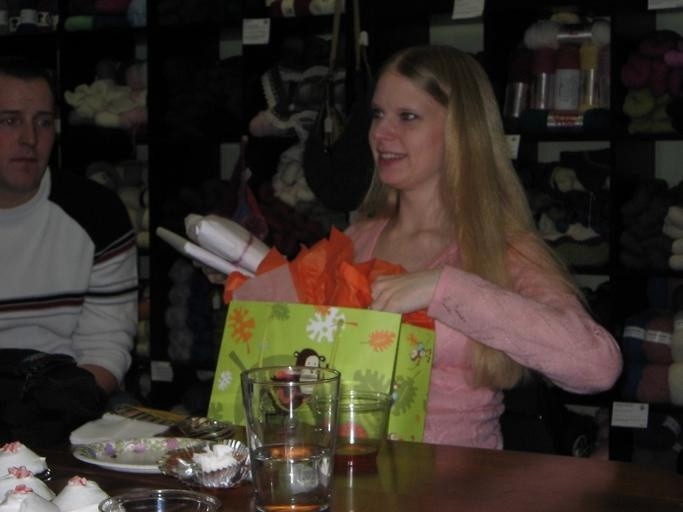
47,2,682,475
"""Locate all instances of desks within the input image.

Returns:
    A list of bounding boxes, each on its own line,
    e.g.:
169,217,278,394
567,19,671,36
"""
27,413,681,512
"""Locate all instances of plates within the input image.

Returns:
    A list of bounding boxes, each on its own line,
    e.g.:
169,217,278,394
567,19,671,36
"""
72,437,206,476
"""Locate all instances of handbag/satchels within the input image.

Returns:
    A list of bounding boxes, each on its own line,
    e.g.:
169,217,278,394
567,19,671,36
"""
206,299,436,442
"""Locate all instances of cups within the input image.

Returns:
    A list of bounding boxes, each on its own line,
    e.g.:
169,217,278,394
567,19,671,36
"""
239,366,395,512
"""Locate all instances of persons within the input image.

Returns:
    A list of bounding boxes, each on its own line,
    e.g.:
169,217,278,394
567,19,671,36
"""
343,45,624,451
0,32,140,408
